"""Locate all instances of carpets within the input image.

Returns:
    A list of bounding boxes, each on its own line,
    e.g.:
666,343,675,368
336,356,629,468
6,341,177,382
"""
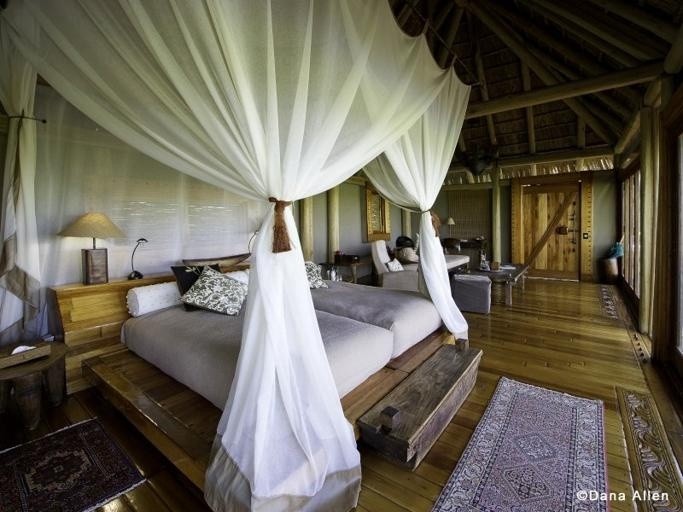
430,376,608,512
0,419,146,511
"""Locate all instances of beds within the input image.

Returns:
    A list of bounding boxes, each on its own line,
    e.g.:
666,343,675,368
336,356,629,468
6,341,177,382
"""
311,280,443,358
120,295,394,413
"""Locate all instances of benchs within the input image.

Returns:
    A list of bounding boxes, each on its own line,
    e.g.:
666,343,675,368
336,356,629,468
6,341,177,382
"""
357,339,484,472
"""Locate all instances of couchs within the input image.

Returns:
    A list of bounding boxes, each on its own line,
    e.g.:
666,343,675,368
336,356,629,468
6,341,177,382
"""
371,240,418,291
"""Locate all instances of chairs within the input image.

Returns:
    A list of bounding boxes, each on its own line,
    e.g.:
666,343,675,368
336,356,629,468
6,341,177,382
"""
442,238,461,254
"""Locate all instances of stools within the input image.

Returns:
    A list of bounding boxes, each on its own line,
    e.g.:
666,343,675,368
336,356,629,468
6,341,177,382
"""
451,274,491,313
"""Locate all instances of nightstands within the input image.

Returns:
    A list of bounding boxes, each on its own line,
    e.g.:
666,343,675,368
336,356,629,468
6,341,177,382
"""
0,338,68,433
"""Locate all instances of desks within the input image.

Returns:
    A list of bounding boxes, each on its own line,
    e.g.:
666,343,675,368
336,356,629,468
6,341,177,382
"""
335,256,372,283
460,240,487,266
443,254,470,274
464,264,530,305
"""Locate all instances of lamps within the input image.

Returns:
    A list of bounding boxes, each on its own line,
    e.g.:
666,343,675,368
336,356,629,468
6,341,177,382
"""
57,213,127,285
127,237,149,280
446,218,455,238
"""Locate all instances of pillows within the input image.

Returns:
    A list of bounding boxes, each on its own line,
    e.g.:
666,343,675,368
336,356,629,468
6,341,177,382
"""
171,263,219,311
245,261,316,275
305,263,328,288
387,258,404,271
178,264,248,315
126,270,248,317
